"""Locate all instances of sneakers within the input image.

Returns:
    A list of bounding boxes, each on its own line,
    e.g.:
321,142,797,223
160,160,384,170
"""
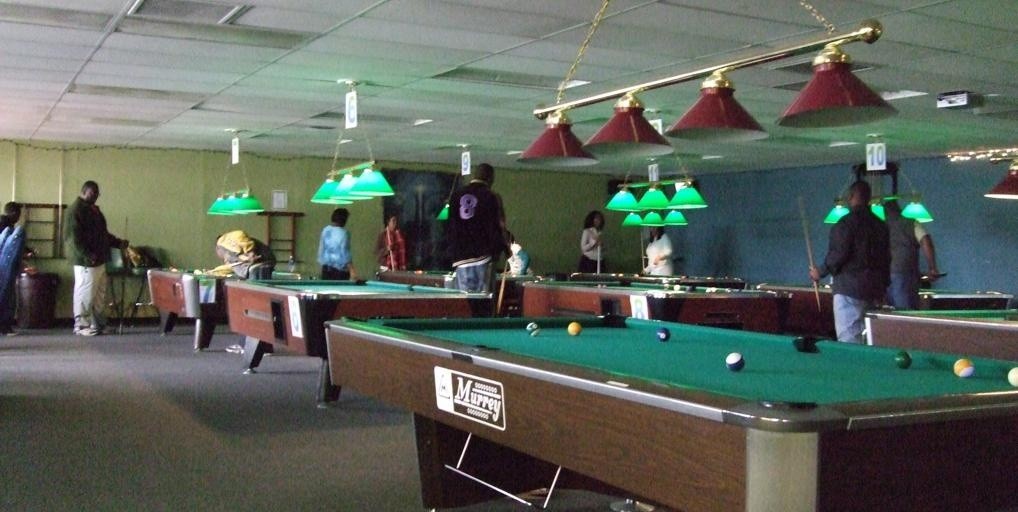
226,342,246,355
262,345,274,357
89,323,117,333
73,325,100,337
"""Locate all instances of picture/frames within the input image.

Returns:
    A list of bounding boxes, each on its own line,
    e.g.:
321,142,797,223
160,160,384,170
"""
270,190,287,211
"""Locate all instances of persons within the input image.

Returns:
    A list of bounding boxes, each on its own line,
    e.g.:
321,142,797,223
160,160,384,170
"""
809,182,892,345
206,231,277,356
431,162,673,317
883,201,939,311
64,181,129,337
375,214,408,272
317,209,358,281
0,202,33,336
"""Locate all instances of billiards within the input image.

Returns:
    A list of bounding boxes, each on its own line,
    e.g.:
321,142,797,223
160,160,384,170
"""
568,322,582,336
726,353,744,371
168,266,194,273
526,322,540,336
408,270,432,275
687,286,695,291
448,271,453,275
598,284,607,288
634,273,639,278
657,328,669,342
1007,367,1018,387
662,279,670,288
894,350,911,368
953,358,974,377
673,284,680,290
194,269,201,275
611,273,624,277
705,287,716,292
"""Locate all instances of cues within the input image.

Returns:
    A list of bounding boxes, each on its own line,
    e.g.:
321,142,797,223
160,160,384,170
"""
597,232,601,274
798,196,821,312
921,272,946,281
119,216,127,335
202,255,261,274
641,230,645,270
496,220,515,314
386,226,395,273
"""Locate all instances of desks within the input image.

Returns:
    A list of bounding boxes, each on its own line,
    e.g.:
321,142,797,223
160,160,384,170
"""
148,262,367,353
325,314,1018,512
377,263,1016,365
225,280,497,406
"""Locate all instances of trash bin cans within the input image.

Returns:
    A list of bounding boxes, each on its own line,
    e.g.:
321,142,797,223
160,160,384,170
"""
15,272,58,330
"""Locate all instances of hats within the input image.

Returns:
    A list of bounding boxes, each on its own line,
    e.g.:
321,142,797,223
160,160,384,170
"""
4,201,24,214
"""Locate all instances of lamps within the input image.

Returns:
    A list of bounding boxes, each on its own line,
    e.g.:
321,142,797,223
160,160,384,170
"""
514,1,916,180
621,209,688,228
819,165,935,225
306,80,395,206
603,137,707,213
983,157,1018,199
206,132,265,216
432,165,462,222
